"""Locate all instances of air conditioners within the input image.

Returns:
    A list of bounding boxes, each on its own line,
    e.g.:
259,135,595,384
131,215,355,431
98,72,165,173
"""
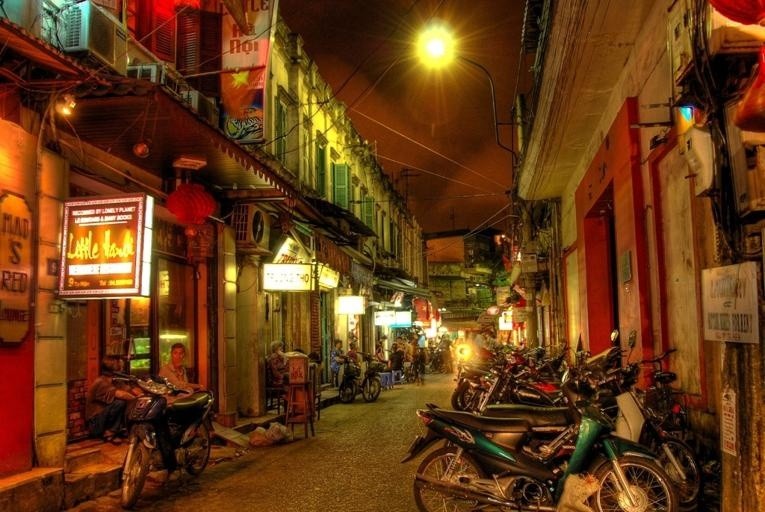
58,0,129,77
128,61,219,129
230,204,272,256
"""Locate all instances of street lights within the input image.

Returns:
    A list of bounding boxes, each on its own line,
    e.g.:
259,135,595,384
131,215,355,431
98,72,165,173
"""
415,25,531,243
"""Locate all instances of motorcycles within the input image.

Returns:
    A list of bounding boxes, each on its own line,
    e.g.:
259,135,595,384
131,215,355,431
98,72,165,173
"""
339,352,385,403
111,371,216,509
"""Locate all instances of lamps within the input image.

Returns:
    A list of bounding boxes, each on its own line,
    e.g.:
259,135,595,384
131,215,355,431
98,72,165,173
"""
133,137,155,161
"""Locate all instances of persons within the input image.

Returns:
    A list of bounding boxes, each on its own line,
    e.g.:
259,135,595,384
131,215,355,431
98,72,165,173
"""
86,359,142,441
158,342,216,440
270,340,290,387
376,336,426,386
331,339,346,373
440,334,455,373
347,342,358,362
439,335,445,345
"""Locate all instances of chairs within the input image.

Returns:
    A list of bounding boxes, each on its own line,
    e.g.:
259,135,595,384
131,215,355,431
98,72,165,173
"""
264,355,321,419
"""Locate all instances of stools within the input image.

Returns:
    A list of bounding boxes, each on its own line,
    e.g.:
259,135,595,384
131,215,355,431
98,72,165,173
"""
379,370,402,390
